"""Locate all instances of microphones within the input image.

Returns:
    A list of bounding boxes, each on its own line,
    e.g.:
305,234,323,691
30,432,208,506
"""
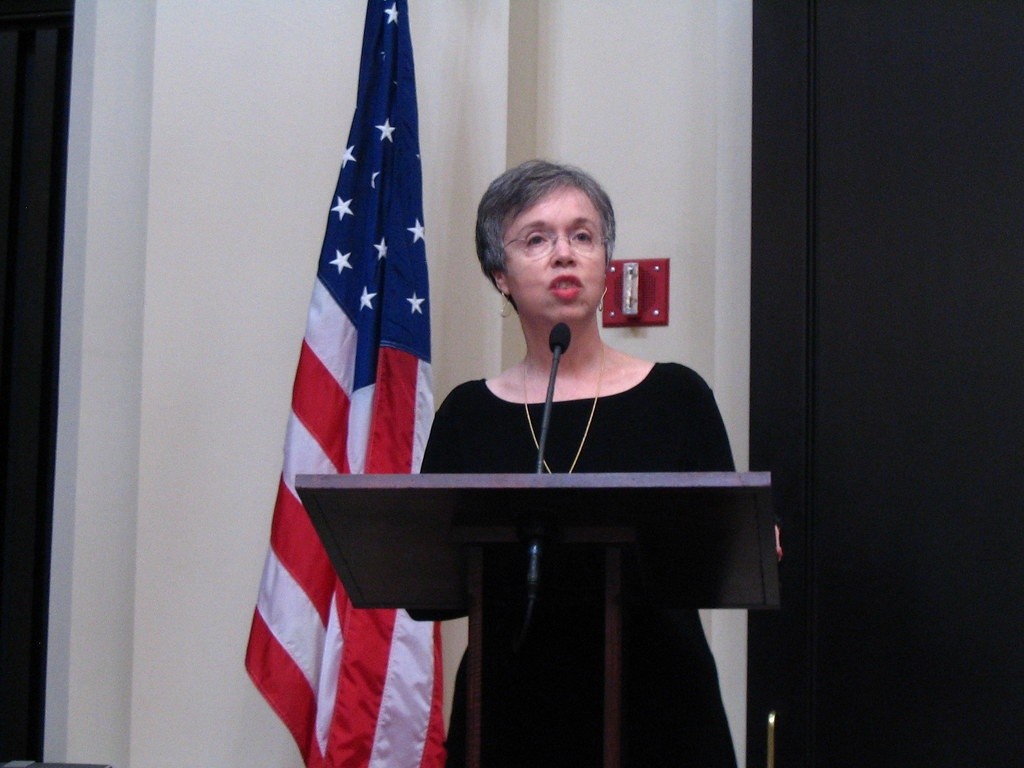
536,321,571,474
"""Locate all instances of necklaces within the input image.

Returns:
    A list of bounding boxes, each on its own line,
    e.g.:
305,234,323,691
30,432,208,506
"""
522,344,605,474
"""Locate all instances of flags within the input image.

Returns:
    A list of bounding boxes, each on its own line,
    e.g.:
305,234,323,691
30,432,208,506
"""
245,0,448,768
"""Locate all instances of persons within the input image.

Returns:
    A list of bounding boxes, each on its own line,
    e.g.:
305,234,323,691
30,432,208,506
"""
403,159,784,768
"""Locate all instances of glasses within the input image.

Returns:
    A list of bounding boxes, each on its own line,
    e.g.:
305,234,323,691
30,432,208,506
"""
500,223,610,257
